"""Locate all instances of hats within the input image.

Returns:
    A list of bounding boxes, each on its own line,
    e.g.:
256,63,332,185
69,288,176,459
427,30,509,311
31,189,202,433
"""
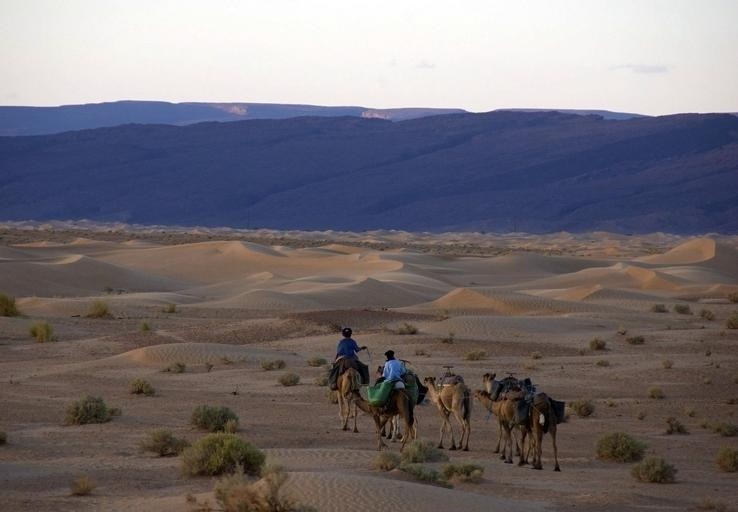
342,328,352,337
384,350,394,356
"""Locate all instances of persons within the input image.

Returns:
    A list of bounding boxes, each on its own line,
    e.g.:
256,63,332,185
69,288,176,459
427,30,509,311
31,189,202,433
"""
334,327,367,359
379,350,406,381
